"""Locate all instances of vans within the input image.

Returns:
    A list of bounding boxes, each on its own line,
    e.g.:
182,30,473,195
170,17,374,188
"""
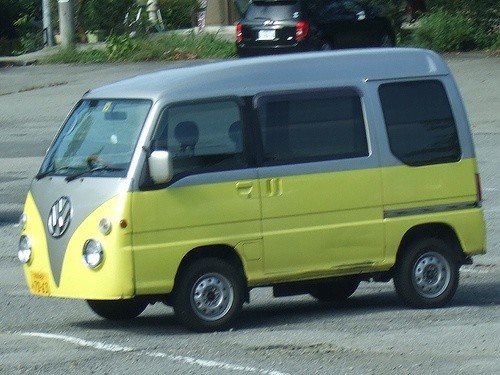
17,46,487,332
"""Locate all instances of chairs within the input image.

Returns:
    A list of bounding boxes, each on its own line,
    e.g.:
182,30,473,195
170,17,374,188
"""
174,120,199,168
228,120,244,170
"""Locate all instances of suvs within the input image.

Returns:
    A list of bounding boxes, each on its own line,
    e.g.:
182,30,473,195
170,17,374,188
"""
236,0,378,57
